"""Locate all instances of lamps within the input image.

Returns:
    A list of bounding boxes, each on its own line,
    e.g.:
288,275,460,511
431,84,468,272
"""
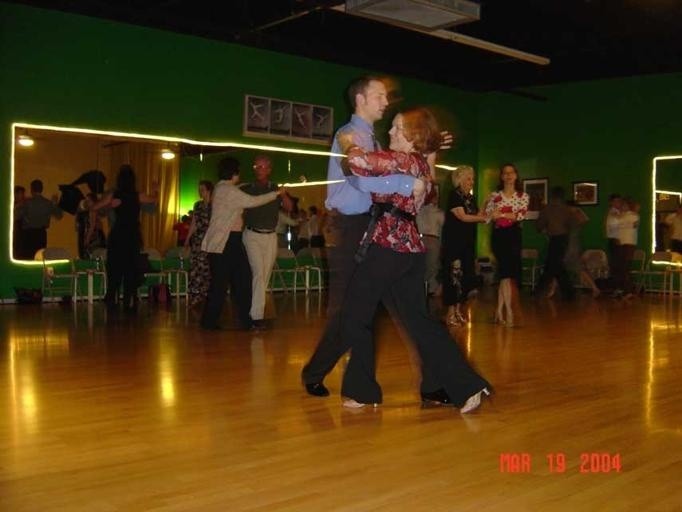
17,128,34,147
160,140,176,161
330,0,479,33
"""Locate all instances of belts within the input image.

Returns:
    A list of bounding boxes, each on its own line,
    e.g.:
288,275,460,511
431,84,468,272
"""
246,226,275,234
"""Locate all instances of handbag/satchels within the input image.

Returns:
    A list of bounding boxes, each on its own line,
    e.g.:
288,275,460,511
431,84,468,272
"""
149,284,168,302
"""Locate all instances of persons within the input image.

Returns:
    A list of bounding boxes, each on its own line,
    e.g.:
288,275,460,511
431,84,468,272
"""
276,188,327,257
199,157,285,330
173,215,191,247
13,178,106,260
332,105,491,415
441,160,486,327
664,203,681,254
184,180,214,309
481,164,529,327
298,74,451,406
527,186,643,302
239,155,294,329
415,188,447,299
655,212,668,252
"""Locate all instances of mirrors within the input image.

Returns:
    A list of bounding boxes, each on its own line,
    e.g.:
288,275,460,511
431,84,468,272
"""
8,124,471,267
653,156,682,266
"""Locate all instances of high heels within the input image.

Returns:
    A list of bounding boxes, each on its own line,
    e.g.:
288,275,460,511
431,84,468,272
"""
460,385,491,414
342,399,377,408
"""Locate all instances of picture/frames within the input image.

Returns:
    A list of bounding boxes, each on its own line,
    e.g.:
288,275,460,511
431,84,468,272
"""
571,181,599,206
521,178,548,221
243,94,333,147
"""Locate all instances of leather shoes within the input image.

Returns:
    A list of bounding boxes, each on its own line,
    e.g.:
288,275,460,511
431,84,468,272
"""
420,388,457,406
250,315,265,328
306,383,329,396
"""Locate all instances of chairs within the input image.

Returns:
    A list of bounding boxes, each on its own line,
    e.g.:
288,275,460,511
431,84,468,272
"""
39,246,329,302
519,246,674,300
